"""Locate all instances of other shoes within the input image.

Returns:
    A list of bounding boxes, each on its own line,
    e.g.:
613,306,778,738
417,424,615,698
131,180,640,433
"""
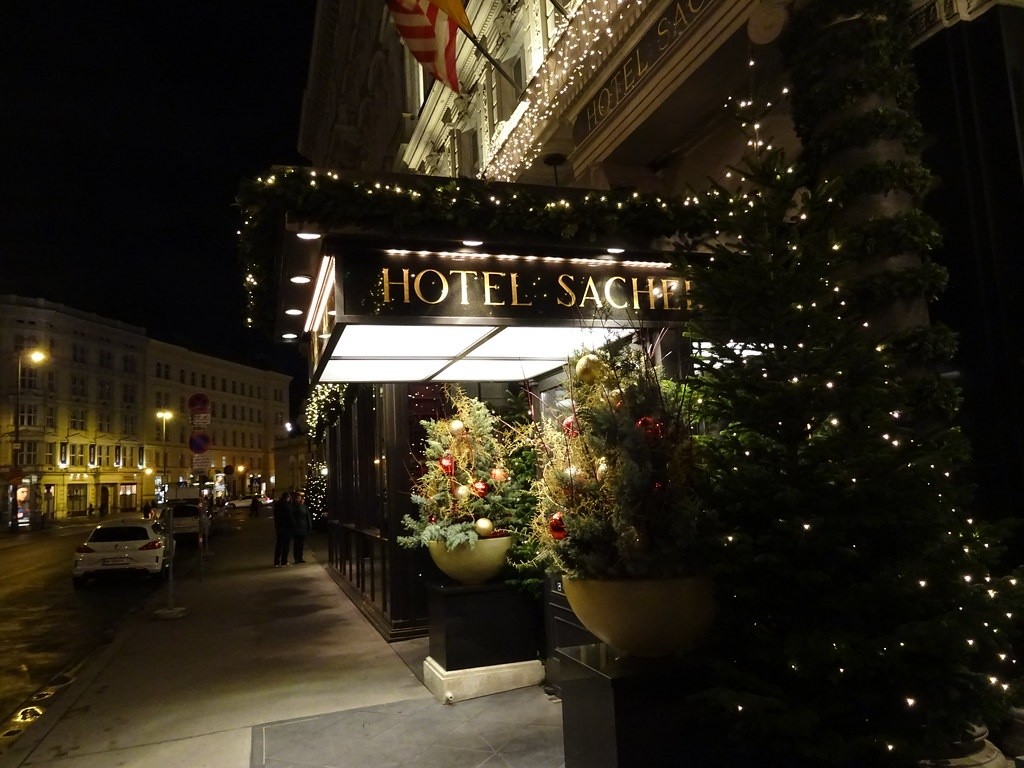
295,561,305,563
281,563,293,567
273,565,279,568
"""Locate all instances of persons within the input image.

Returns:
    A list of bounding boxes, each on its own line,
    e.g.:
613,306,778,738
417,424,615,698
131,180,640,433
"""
151,500,158,519
143,502,151,519
291,493,312,565
87,503,93,519
15,483,29,520
248,495,260,518
273,491,294,568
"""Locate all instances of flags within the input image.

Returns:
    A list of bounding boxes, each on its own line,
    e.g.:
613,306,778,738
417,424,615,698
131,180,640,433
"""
386,0,476,96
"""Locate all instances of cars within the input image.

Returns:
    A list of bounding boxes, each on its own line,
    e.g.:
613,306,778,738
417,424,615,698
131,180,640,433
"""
225,496,253,510
160,503,212,535
70,517,177,589
254,494,270,504
148,498,205,519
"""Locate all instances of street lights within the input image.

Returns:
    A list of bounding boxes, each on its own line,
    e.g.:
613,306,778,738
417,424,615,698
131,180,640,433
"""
8,345,46,534
156,408,172,476
